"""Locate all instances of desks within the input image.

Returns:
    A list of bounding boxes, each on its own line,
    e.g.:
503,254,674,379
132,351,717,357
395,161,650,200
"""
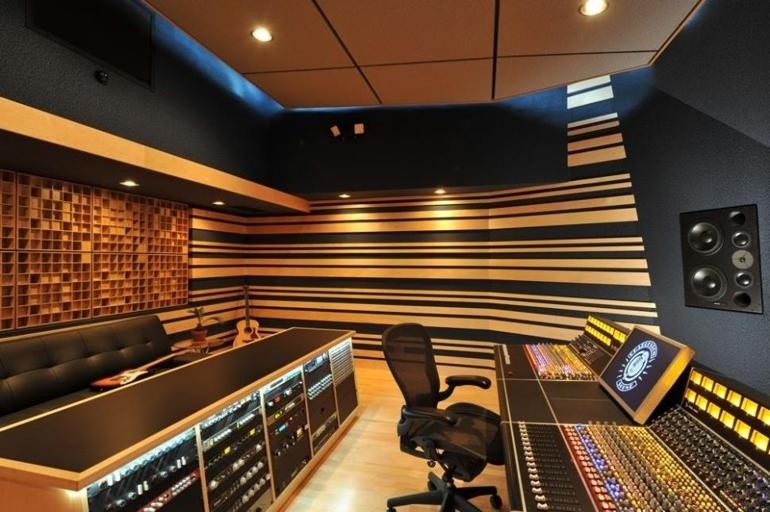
174,339,225,357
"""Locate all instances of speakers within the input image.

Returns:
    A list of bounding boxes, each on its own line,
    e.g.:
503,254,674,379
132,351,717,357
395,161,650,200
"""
678,203,764,315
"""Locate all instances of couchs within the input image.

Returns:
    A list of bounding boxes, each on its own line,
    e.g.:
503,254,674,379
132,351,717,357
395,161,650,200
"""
1,315,213,428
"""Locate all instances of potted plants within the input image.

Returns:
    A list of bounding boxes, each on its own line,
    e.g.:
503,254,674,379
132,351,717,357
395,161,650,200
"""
187,304,222,341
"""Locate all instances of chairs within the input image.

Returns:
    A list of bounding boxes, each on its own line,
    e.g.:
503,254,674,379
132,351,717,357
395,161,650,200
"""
381,323,506,511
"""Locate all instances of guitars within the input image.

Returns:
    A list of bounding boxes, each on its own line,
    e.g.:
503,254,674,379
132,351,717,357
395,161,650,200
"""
232,281,263,349
89,347,210,390
